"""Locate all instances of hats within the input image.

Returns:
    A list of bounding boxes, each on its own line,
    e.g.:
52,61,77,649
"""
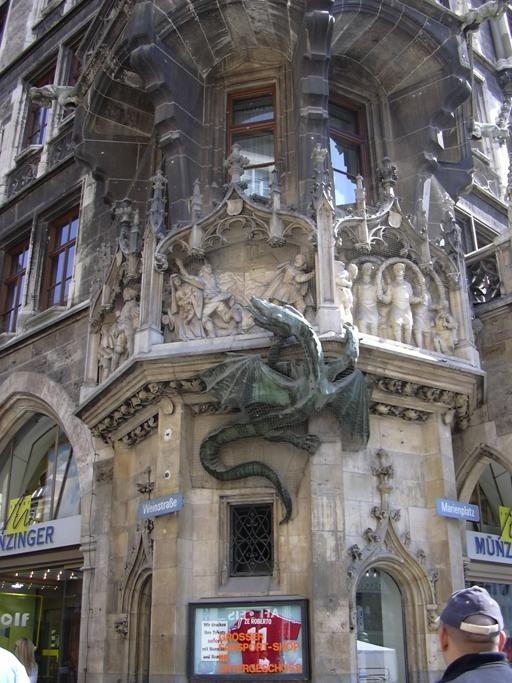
438,586,504,635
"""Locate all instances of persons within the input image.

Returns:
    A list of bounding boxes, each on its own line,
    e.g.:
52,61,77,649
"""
13,636,40,683
380,260,426,344
434,584,511,682
0,646,31,683
263,253,317,317
98,286,141,381
335,262,360,331
353,262,385,336
164,253,244,340
